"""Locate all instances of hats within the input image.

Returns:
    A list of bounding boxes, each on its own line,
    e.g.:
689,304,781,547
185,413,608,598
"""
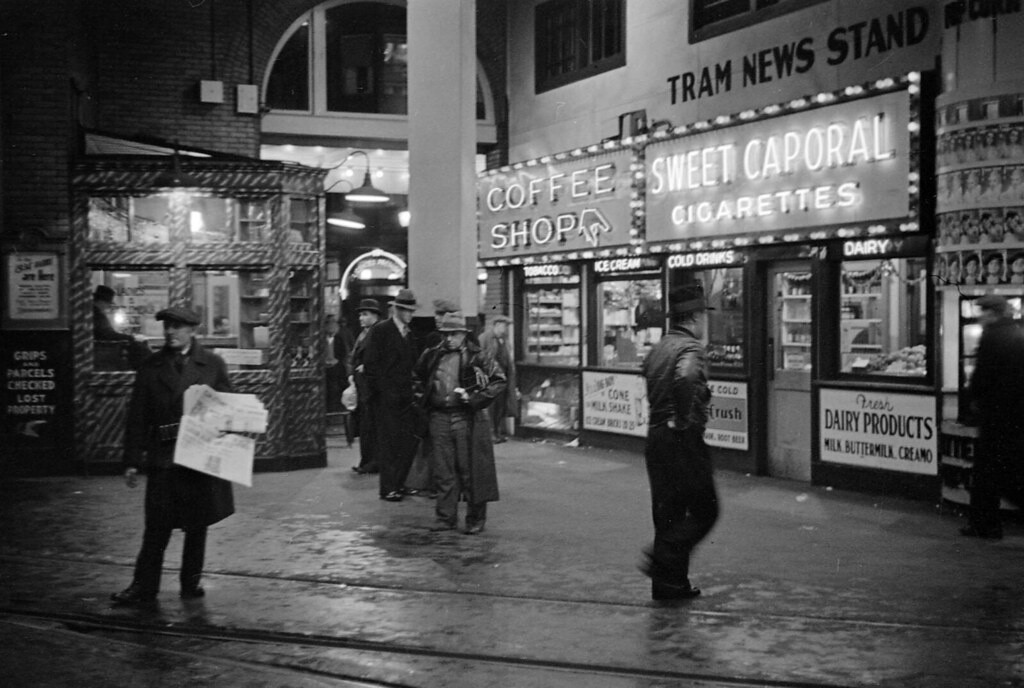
155,306,201,325
437,312,468,332
355,299,381,313
92,285,115,301
387,290,422,310
492,315,514,324
662,284,716,317
974,295,1007,312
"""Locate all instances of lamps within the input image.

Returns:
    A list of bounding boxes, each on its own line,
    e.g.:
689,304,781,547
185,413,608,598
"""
316,150,390,204
321,180,365,230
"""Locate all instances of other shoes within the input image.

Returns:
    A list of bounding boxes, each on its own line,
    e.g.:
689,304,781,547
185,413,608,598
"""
359,467,378,473
381,490,402,501
430,519,458,531
398,486,418,495
352,466,359,470
463,520,485,533
110,584,157,602
637,552,653,574
958,524,1002,539
180,585,205,599
652,587,700,600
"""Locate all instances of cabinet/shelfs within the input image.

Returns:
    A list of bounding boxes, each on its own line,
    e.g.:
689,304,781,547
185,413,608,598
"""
239,294,311,325
527,288,563,347
781,293,883,349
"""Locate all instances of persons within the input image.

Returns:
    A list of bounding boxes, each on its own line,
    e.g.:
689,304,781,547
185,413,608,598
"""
109,306,238,604
352,289,430,500
1003,210,1024,243
1007,251,1023,284
959,213,978,245
936,128,1024,168
979,213,1001,244
93,286,153,359
981,252,1004,284
345,298,384,476
632,283,719,605
963,168,1024,207
953,293,1024,541
213,315,231,336
961,253,980,284
477,316,518,445
946,254,960,285
944,217,958,244
411,300,508,534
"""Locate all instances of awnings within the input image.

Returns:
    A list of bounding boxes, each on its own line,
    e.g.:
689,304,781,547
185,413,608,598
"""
260,142,487,206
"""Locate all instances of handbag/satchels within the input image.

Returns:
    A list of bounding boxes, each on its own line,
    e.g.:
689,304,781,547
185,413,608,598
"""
341,384,358,411
